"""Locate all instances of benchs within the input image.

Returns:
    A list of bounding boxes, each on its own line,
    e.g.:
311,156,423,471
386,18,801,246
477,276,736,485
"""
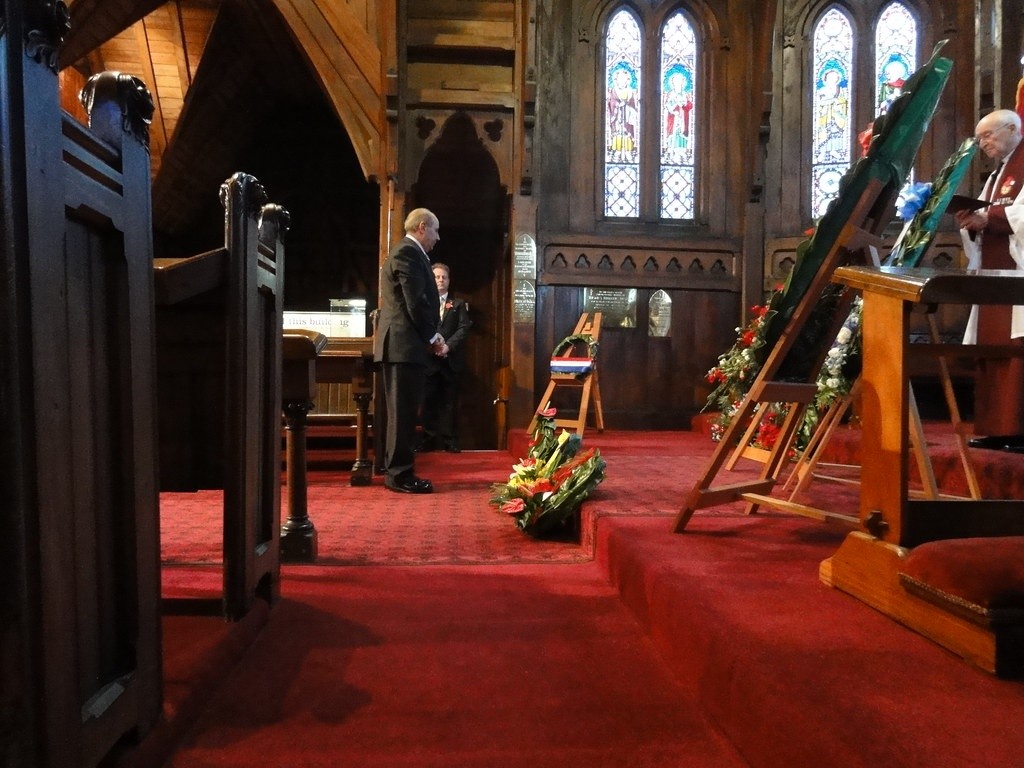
280,350,381,487
0,0,293,768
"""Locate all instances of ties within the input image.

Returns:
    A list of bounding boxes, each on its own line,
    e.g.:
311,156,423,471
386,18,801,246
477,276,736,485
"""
440,298,445,318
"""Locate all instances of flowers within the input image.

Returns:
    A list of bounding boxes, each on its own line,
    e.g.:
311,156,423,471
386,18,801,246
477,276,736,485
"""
489,402,606,533
696,111,933,467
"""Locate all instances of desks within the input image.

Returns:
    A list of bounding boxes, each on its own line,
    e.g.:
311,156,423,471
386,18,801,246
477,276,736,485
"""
820,266,1023,677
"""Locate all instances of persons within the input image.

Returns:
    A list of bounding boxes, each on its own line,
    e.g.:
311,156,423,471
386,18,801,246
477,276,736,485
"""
373,208,445,493
413,262,473,453
954,110,1024,452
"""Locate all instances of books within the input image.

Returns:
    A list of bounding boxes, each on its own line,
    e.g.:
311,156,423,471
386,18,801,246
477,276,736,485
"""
945,195,992,214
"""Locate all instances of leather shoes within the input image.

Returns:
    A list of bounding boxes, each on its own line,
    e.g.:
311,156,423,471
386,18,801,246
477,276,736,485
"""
385,476,433,493
969,435,1024,453
414,440,435,452
441,443,461,453
